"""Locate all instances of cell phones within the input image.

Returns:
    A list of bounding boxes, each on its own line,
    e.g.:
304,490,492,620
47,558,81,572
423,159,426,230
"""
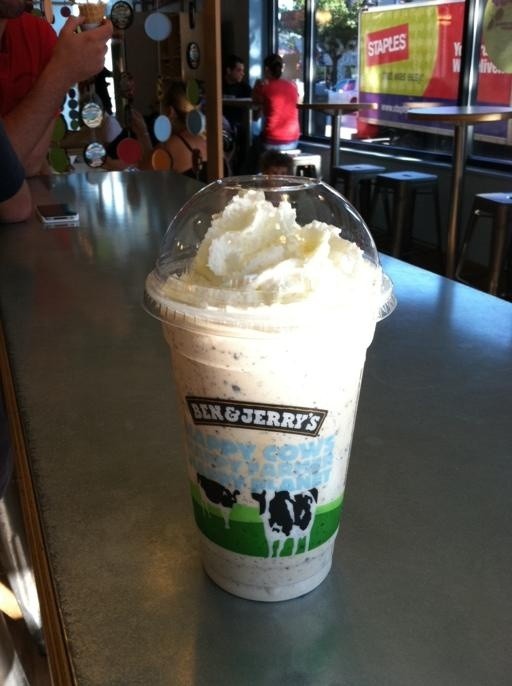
35,202,79,223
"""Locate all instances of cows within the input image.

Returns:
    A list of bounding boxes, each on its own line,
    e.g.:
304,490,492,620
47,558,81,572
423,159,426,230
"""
190,469,240,529
251,487,318,558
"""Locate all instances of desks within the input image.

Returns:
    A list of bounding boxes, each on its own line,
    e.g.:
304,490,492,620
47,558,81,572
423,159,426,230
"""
297,102,379,189
230,98,269,163
398,106,511,277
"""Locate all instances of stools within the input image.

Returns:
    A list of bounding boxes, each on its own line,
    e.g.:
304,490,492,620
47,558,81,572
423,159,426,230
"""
264,148,511,299
